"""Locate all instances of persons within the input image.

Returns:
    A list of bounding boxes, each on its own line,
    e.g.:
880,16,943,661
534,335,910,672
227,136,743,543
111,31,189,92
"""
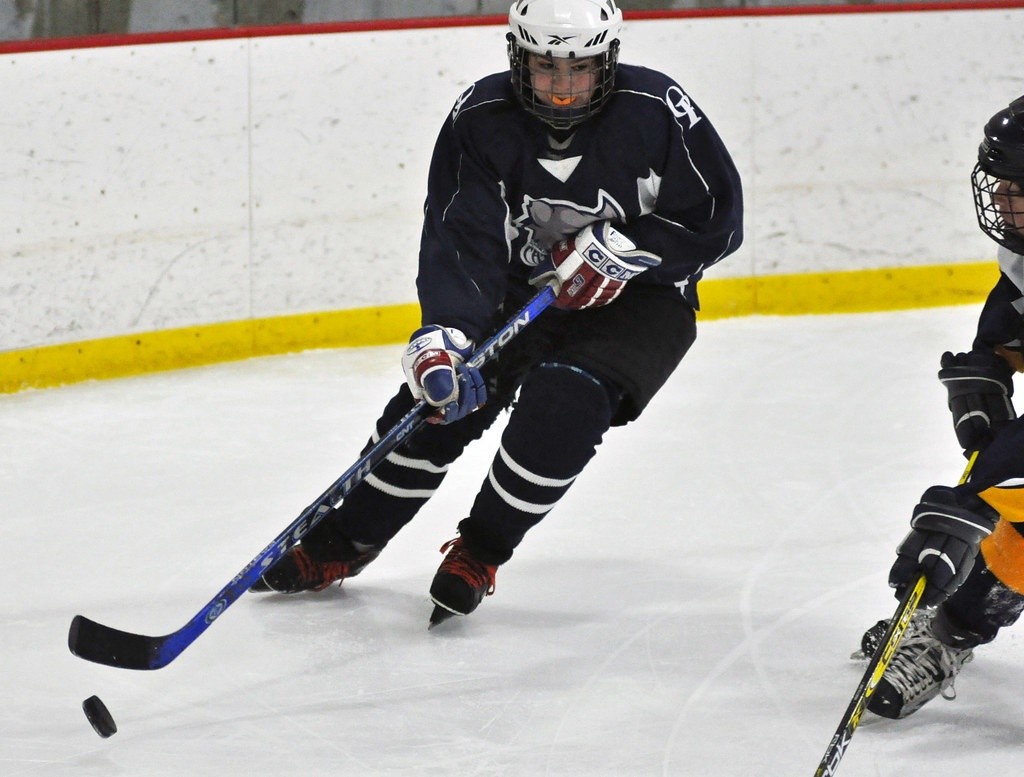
853,96,1024,723
250,0,743,631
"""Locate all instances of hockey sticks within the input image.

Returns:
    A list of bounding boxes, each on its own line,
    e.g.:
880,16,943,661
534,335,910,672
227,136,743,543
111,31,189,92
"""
66,277,559,671
811,442,978,777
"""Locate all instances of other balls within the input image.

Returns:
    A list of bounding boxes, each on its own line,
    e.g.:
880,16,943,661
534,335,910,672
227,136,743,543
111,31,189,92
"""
82,695,117,738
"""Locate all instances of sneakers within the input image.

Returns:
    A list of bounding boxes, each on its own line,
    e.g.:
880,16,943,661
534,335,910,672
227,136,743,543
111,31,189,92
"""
849,606,997,727
429,518,512,629
246,508,387,594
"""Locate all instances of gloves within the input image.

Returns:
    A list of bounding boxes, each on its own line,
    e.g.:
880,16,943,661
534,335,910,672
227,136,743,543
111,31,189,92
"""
938,350,1024,488
528,220,663,310
401,323,488,425
888,486,1000,610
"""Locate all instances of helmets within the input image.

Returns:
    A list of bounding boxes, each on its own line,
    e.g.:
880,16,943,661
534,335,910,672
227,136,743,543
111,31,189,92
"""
970,95,1024,257
506,0,623,129
